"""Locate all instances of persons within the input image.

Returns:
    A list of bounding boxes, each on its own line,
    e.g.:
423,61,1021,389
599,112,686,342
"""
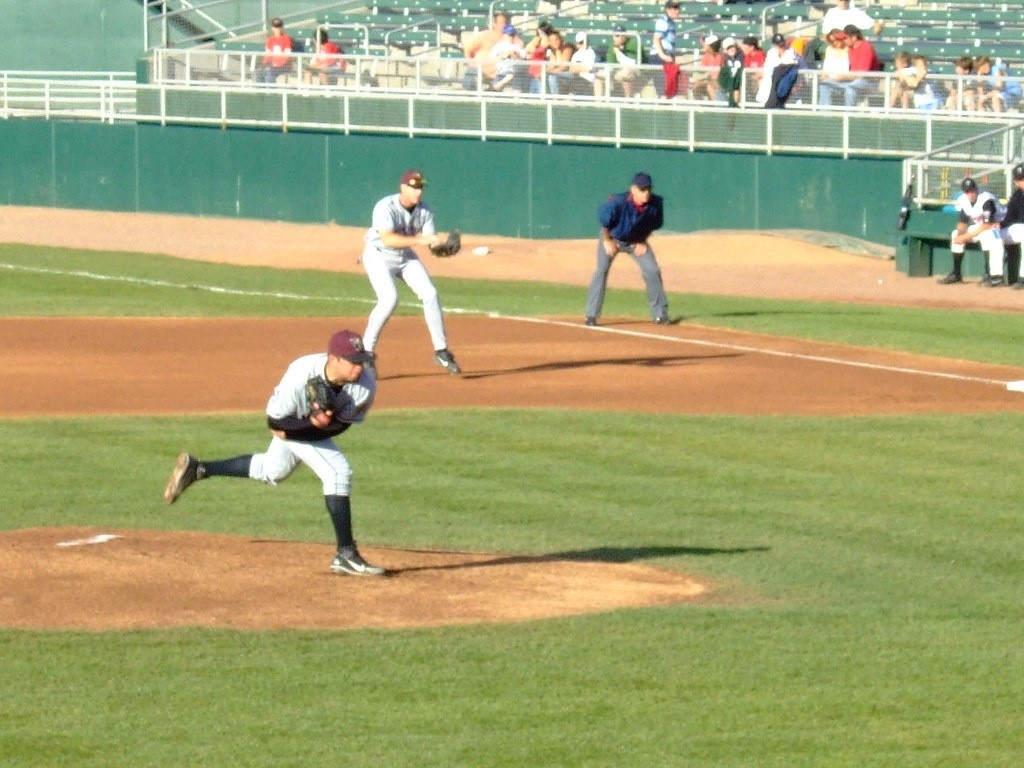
363,171,461,380
693,33,807,103
938,166,1024,288
305,31,345,84
890,52,941,116
819,0,882,111
256,19,294,89
650,3,680,98
946,56,1022,119
584,174,672,326
462,14,644,97
164,330,386,576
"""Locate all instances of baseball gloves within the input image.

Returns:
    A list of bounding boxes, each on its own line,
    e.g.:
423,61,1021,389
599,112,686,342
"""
431,230,460,256
305,376,336,416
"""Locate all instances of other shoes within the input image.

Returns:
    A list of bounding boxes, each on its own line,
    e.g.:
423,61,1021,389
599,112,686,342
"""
983,277,1004,287
942,274,962,283
655,316,671,324
585,316,596,326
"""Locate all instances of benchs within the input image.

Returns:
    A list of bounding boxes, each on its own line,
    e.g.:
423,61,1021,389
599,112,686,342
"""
884,229,1021,287
213,0,1024,106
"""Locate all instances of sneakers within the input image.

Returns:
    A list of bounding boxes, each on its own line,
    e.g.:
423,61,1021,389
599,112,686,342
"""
331,548,385,576
436,350,459,373
165,452,203,503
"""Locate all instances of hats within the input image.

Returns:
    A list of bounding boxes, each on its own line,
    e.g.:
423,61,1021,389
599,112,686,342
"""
772,34,786,45
836,25,858,39
633,172,653,190
503,25,517,36
402,172,428,188
615,26,625,32
962,179,975,190
722,38,737,50
1014,165,1024,180
666,0,683,9
329,329,376,364
272,18,282,26
